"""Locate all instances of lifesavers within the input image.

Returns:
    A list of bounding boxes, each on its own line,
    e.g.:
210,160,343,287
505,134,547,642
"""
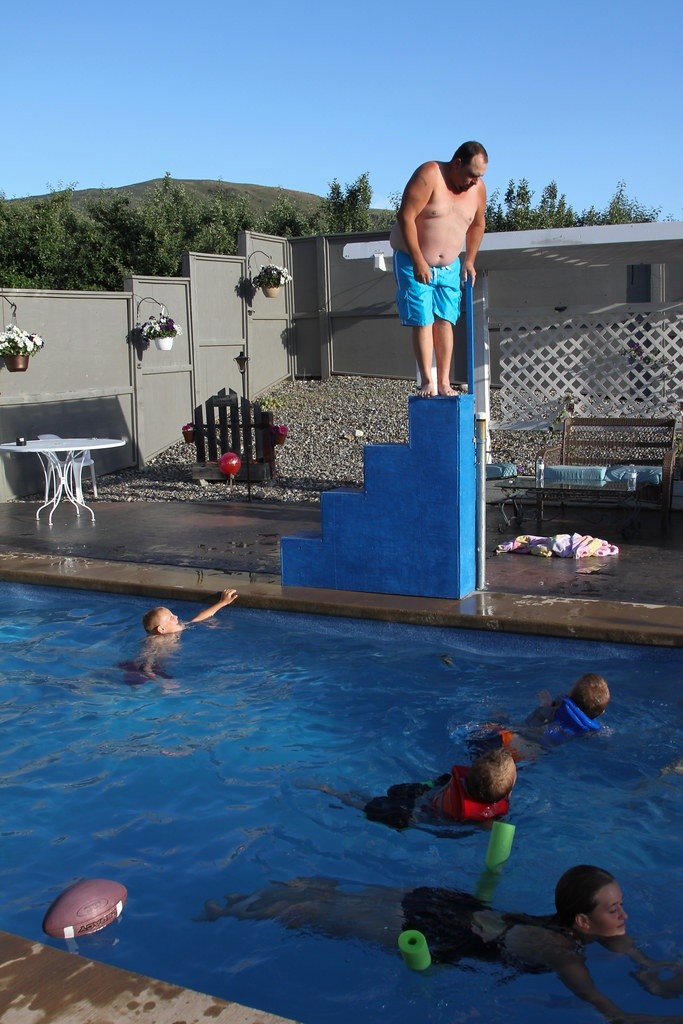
397,818,518,972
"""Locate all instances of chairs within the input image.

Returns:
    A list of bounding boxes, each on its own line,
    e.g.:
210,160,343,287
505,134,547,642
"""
38,434,97,502
192,388,277,482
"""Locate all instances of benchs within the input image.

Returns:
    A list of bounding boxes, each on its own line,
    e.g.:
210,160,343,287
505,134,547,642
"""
486,418,678,530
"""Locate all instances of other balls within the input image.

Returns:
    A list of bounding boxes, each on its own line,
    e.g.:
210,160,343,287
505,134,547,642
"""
217,452,242,474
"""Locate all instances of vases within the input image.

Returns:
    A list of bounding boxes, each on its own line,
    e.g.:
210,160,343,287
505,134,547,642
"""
182,432,194,443
155,337,174,350
275,434,287,444
4,355,29,372
262,285,279,297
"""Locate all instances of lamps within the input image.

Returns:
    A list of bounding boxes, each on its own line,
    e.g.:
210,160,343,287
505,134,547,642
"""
234,352,251,374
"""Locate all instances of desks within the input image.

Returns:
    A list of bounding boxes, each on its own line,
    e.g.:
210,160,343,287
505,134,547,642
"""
493,478,646,540
0,438,126,525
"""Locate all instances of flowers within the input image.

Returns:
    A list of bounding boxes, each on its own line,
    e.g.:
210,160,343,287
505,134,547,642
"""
273,425,288,436
141,314,182,339
253,264,292,287
0,324,44,357
182,422,194,432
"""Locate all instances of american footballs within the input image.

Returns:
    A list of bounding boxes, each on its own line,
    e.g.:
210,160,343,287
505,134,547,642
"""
42,877,128,939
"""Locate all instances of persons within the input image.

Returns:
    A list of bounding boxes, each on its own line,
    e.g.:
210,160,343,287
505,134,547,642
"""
192,861,683,1024
309,751,517,838
118,588,257,689
468,672,640,769
389,140,487,398
